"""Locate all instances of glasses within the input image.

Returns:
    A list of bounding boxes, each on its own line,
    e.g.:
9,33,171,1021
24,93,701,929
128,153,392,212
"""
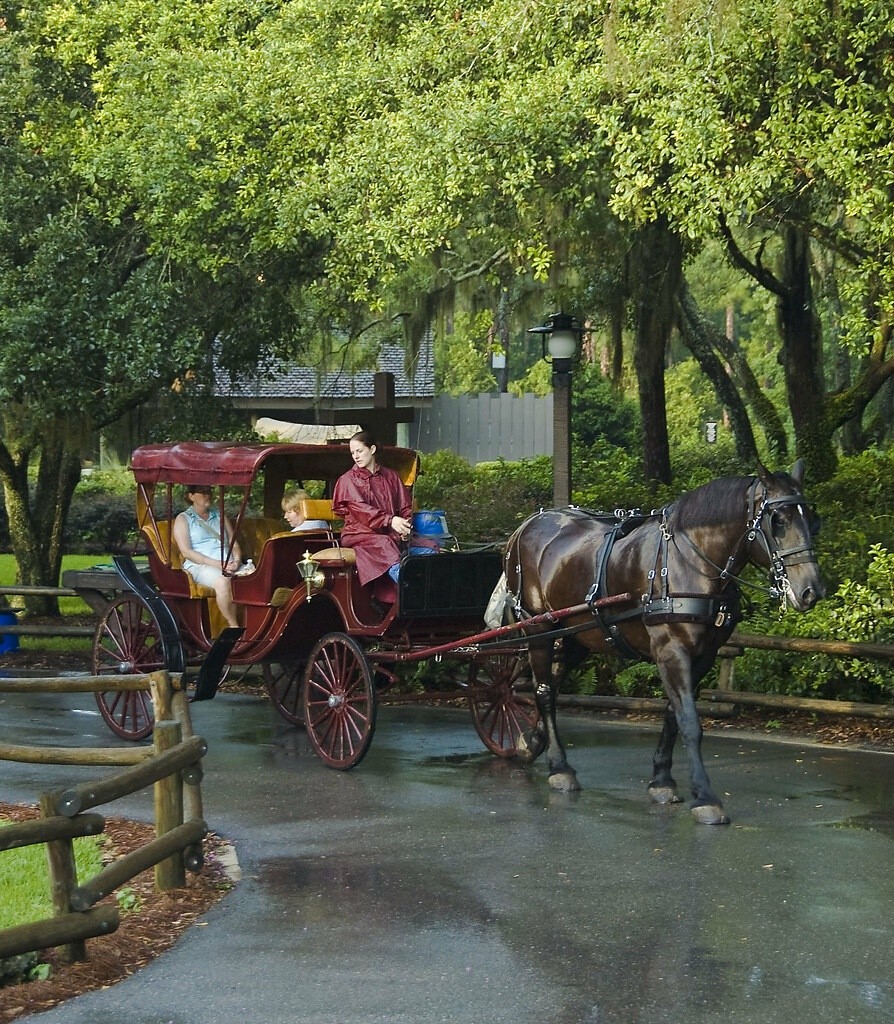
195,490,212,494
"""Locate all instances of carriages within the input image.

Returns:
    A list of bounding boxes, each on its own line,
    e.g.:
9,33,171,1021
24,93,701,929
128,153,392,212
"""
59,441,829,825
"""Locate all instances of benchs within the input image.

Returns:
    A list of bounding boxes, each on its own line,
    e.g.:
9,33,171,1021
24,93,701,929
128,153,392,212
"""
144,518,284,640
300,499,357,566
230,528,337,640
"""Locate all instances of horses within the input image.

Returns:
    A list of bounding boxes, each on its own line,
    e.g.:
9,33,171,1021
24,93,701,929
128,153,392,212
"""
506,459,821,824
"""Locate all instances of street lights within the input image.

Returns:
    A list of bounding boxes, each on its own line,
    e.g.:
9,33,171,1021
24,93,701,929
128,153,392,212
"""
525,313,600,508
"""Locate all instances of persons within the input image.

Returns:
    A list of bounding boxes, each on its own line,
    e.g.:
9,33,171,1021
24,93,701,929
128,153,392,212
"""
332,430,438,583
281,490,328,531
174,484,246,628
